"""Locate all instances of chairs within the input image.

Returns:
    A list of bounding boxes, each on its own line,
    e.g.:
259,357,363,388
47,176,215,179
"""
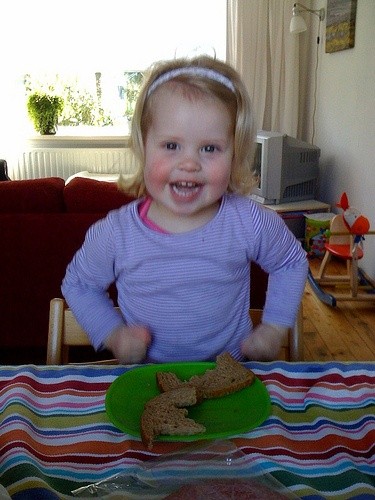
47,298,304,366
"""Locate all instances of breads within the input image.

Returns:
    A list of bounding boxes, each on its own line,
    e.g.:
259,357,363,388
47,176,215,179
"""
141,351,255,450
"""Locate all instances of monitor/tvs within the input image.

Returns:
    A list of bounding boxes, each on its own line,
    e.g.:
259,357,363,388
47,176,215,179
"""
249,130,321,205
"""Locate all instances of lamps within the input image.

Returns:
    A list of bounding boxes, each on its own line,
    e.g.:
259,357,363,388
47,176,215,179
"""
289,3,326,33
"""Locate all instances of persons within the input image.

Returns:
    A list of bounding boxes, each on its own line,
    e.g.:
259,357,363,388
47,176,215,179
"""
60,47,311,364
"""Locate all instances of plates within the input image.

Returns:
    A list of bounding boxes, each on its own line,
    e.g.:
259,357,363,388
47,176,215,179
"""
105,362,271,442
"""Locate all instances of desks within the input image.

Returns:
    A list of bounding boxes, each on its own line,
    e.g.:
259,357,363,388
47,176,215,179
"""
0,358,375,500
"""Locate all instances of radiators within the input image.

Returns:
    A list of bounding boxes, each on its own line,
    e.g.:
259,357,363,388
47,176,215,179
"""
7,147,139,182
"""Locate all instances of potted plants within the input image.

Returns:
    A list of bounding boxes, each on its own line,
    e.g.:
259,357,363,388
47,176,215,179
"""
27,85,64,136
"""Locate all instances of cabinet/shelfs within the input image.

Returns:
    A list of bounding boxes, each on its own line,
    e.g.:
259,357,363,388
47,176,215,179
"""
262,199,331,242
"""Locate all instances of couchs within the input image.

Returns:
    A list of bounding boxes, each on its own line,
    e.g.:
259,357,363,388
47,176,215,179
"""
0,178,139,345
